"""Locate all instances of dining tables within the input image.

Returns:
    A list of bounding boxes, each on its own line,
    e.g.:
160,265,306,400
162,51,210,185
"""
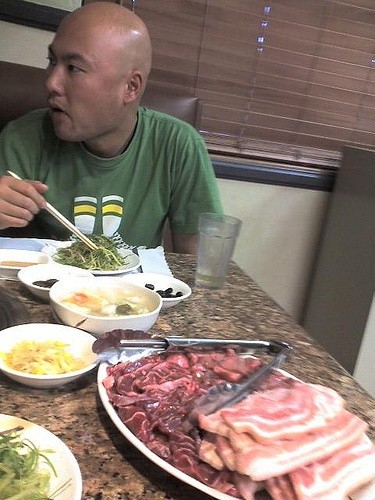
0,251,375,500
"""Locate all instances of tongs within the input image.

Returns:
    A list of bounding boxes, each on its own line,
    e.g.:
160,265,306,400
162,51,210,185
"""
90,327,290,433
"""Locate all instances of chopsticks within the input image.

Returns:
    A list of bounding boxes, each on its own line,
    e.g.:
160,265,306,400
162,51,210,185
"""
6,169,98,251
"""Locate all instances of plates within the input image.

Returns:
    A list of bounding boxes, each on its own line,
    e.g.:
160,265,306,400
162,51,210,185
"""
98,345,374,500
43,240,140,274
0,411,83,500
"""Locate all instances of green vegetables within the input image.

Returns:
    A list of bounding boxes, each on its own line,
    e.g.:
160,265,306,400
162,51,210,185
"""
53,233,128,271
0,426,72,500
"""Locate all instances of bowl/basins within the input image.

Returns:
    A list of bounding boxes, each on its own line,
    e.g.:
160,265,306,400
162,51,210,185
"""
17,264,96,301
119,272,191,313
0,322,103,389
46,276,163,337
0,248,49,278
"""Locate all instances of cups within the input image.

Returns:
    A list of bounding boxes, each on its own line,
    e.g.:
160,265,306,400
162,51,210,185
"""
195,212,241,290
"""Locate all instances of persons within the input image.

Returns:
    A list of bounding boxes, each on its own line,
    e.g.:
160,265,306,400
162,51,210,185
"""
0,2,223,255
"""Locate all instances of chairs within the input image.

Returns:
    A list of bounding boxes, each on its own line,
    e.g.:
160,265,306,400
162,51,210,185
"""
0,59,203,252
